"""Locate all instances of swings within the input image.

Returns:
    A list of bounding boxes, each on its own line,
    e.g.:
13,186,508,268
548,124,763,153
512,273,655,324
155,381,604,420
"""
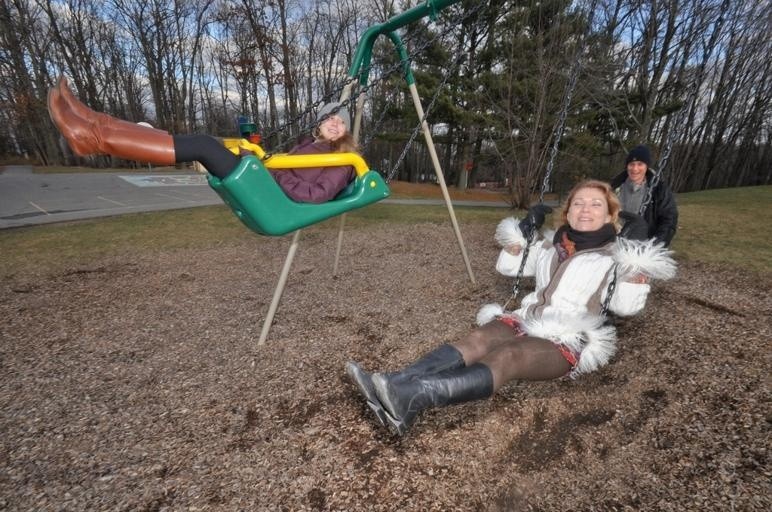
502,0,731,356
206,0,515,237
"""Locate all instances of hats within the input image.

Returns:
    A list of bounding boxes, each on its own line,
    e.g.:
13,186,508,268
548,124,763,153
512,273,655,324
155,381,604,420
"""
317,101,352,134
625,143,651,168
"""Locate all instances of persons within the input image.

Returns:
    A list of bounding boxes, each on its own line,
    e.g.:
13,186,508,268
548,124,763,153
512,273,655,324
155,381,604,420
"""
344,178,677,439
606,144,678,253
46,72,358,205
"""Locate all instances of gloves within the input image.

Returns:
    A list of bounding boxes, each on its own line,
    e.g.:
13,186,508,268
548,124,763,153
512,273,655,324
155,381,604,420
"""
518,205,554,238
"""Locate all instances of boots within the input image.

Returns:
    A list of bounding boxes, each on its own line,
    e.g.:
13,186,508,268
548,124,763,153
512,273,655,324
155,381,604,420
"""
46,72,176,167
344,343,467,428
370,360,496,435
